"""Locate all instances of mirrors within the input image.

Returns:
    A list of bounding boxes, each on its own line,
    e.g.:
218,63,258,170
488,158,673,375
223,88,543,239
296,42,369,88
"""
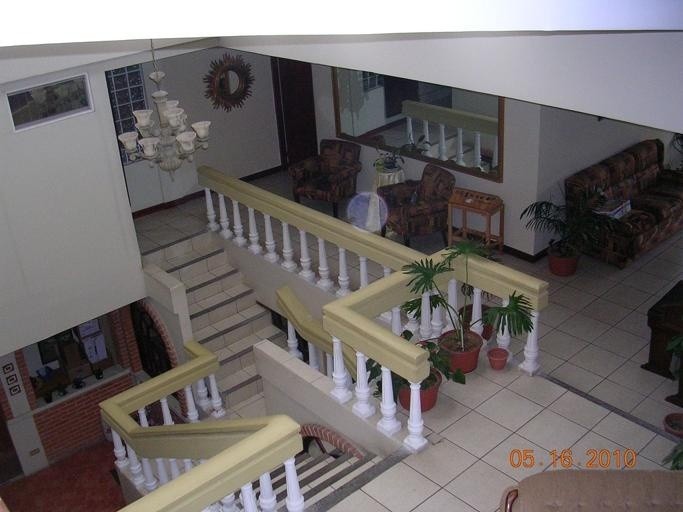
330,65,504,183
202,50,256,114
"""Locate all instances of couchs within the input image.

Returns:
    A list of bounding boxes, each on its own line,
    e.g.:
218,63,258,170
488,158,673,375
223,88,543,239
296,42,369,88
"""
564,138,682,272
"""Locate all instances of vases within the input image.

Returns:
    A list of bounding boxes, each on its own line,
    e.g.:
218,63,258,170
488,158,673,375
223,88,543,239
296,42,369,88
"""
664,412,683,437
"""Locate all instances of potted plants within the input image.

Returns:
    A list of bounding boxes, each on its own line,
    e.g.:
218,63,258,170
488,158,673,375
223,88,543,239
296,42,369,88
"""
518,191,630,275
365,239,535,413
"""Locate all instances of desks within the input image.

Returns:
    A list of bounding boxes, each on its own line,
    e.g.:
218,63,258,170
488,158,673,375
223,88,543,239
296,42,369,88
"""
379,171,406,185
641,280,683,406
448,186,505,256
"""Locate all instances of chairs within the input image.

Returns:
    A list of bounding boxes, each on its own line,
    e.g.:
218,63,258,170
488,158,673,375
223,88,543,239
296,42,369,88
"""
501,468,682,511
286,137,363,217
377,163,456,248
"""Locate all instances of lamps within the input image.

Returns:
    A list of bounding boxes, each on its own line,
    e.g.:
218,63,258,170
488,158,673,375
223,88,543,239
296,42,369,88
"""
119,40,212,182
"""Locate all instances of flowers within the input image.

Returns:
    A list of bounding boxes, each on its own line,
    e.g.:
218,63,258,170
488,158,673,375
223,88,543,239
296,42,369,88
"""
371,143,407,170
